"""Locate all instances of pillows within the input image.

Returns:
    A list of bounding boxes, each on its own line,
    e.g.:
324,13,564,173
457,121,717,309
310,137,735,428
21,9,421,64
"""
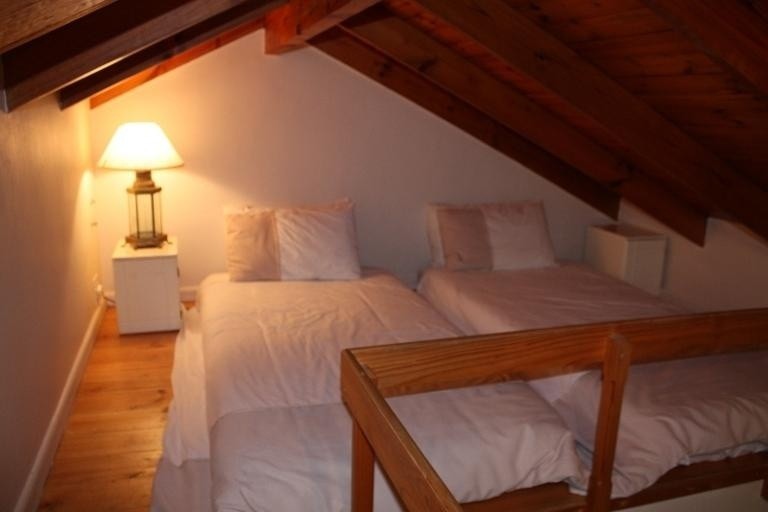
224,199,363,282
428,197,561,266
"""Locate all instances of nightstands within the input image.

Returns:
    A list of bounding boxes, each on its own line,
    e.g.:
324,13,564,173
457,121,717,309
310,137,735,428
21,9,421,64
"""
584,221,670,298
113,235,181,335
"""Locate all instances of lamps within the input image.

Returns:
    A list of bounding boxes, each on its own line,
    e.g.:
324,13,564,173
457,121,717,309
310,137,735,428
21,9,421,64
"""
97,121,184,247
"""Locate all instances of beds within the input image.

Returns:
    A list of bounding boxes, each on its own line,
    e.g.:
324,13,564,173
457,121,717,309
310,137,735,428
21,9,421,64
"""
417,257,767,510
151,264,583,512
